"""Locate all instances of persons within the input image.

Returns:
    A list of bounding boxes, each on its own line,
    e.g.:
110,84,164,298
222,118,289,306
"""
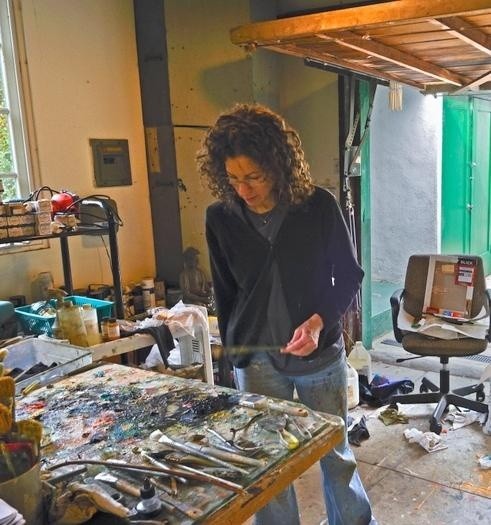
180,247,216,317
195,104,374,525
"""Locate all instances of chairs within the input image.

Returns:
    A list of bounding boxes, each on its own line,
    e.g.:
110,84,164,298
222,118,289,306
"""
390,255,491,436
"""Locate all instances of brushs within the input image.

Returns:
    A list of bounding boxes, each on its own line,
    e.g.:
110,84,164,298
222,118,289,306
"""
240,393,308,417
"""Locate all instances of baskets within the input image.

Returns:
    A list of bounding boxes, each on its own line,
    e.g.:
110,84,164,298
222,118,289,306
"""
13,295,115,337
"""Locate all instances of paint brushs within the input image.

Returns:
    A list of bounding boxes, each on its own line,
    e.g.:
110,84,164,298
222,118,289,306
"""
94,424,268,514
1,348,43,484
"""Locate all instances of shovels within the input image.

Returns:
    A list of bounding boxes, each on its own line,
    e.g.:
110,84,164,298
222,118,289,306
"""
255,414,300,450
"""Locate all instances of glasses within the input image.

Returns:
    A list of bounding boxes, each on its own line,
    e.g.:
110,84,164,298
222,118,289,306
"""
220,172,268,185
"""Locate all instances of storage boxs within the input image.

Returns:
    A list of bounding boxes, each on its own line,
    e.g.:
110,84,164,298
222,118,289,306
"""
2,337,93,395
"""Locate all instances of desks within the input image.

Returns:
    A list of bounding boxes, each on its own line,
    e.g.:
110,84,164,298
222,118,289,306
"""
14,362,347,525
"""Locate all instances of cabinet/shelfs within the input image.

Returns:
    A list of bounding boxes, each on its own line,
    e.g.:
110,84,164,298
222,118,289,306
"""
0,200,129,366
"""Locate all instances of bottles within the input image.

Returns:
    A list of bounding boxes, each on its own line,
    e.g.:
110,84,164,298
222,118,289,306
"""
57,300,120,348
132,276,185,315
347,341,372,386
346,360,359,410
